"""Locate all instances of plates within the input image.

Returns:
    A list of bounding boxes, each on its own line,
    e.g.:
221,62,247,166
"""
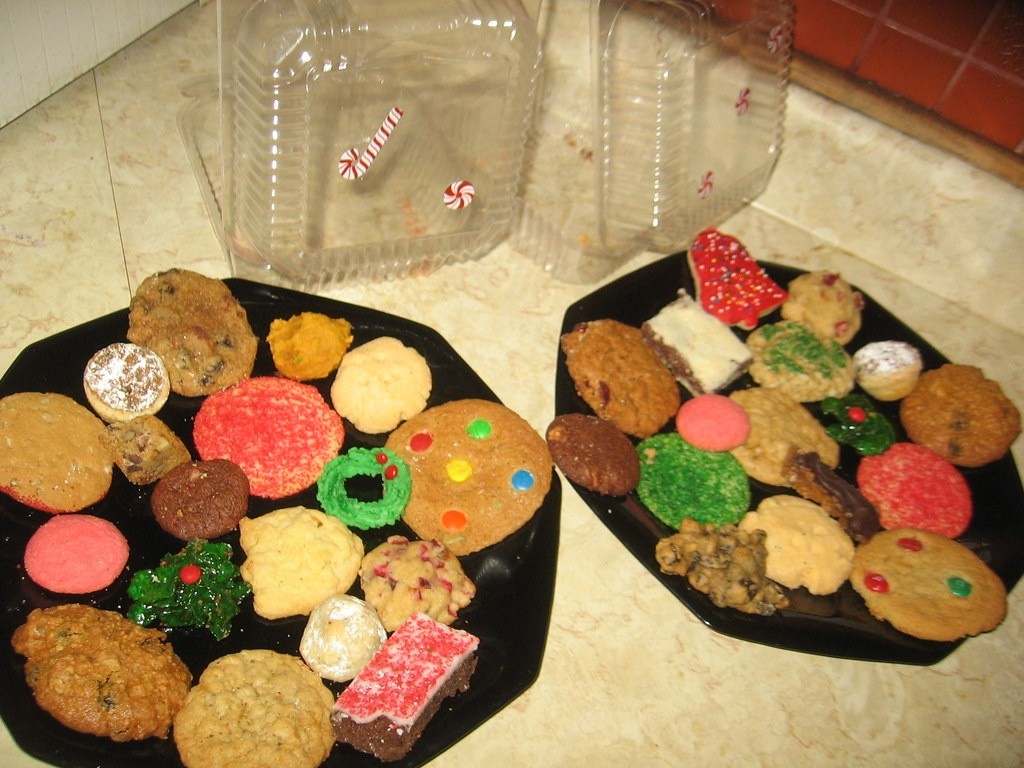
0,278,561,768
555,250,1024,667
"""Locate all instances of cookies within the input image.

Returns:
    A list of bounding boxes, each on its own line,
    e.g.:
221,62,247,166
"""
0,226,1023,768
